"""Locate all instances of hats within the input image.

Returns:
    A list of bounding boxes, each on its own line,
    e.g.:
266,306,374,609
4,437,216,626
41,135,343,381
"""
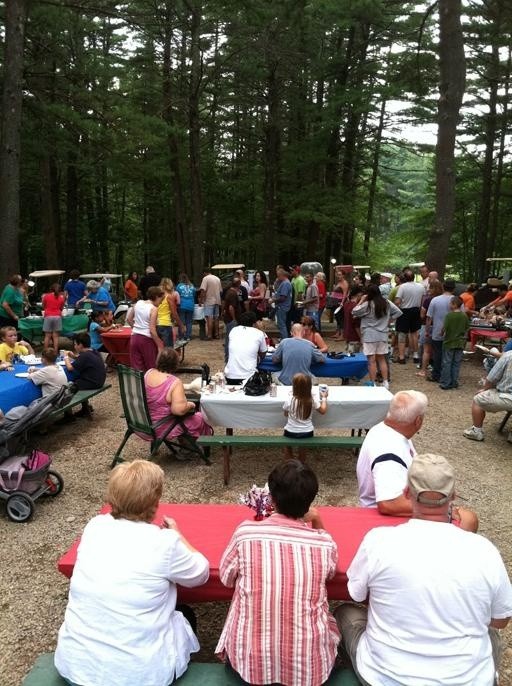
380,273,392,279
289,265,300,272
408,454,456,505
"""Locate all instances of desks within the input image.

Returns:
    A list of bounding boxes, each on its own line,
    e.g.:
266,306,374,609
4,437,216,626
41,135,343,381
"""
58,503,461,602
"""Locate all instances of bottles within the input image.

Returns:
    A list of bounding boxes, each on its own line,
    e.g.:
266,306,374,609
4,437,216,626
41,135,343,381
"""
202,372,228,396
269,382,277,397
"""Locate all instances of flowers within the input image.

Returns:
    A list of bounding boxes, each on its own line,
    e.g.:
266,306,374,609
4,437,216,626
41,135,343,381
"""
240,483,272,514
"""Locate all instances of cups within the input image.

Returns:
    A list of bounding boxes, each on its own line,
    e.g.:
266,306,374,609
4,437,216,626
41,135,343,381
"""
318,384,327,401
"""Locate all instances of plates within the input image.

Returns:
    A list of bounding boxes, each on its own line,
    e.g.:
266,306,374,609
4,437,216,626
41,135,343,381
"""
56,361,66,366
14,373,29,378
25,358,43,365
333,306,342,314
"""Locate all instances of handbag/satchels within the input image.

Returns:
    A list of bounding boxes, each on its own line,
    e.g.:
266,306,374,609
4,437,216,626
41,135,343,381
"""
243,370,272,396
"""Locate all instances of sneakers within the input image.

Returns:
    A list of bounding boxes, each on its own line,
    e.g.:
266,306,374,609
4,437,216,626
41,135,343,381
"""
463,425,484,440
400,352,433,376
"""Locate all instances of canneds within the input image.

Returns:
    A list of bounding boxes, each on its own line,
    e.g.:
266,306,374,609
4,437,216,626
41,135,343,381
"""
209,381,215,393
270,383,277,397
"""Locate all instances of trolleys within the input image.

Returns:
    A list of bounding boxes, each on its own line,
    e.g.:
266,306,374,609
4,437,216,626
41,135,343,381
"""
1,385,63,523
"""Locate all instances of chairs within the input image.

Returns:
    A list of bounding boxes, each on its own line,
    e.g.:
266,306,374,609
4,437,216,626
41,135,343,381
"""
108,364,211,470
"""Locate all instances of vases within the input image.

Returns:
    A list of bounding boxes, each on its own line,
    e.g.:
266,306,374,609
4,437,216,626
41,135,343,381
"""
254,514,263,521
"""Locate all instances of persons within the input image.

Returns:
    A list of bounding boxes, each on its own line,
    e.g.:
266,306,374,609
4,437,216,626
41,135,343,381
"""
2,262,512,465
332,452,512,686
55,461,211,685
353,390,478,538
211,459,343,685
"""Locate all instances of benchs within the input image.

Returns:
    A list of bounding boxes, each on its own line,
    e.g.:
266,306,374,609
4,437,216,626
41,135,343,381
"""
22,654,361,686
196,434,363,485
